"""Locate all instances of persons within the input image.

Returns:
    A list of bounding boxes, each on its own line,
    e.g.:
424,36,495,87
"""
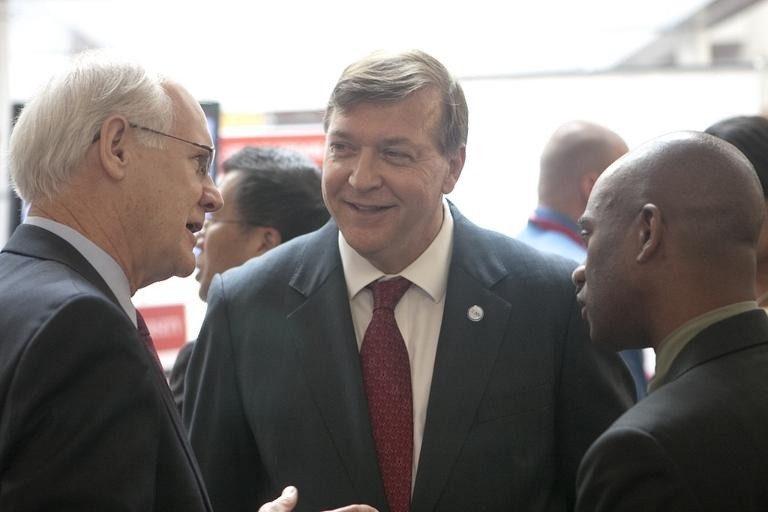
566,126,767,512
177,49,641,510
696,108,767,319
1,50,385,512
163,143,332,426
522,112,651,394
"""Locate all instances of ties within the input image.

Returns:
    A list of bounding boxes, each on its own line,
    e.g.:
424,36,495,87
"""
359,276,411,512
133,307,162,376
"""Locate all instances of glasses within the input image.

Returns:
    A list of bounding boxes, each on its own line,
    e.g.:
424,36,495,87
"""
130,123,216,173
